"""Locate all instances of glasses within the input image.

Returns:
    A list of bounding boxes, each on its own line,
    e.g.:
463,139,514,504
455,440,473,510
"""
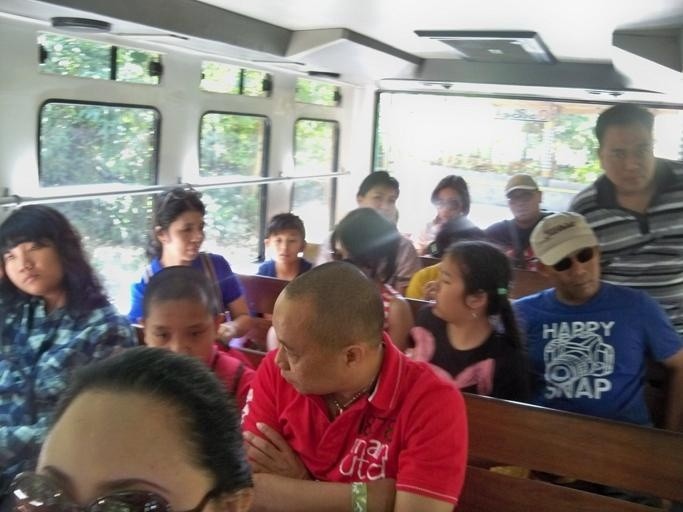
10,472,218,512
156,183,202,217
549,246,595,272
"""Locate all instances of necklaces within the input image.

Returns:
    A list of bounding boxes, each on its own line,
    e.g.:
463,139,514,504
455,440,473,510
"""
330,379,375,414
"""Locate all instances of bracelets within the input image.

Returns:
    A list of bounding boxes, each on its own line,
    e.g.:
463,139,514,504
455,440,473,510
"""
351,481,367,511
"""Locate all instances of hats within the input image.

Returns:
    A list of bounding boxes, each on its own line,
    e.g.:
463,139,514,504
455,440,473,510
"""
504,173,539,195
529,211,600,267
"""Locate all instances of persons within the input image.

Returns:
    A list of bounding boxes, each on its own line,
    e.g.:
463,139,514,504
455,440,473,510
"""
511,211,683,495
248,213,313,318
403,242,534,404
0,205,138,512
568,103,683,337
0,345,255,512
330,207,414,353
236,261,469,512
135,264,257,421
357,171,555,300
127,185,252,349
